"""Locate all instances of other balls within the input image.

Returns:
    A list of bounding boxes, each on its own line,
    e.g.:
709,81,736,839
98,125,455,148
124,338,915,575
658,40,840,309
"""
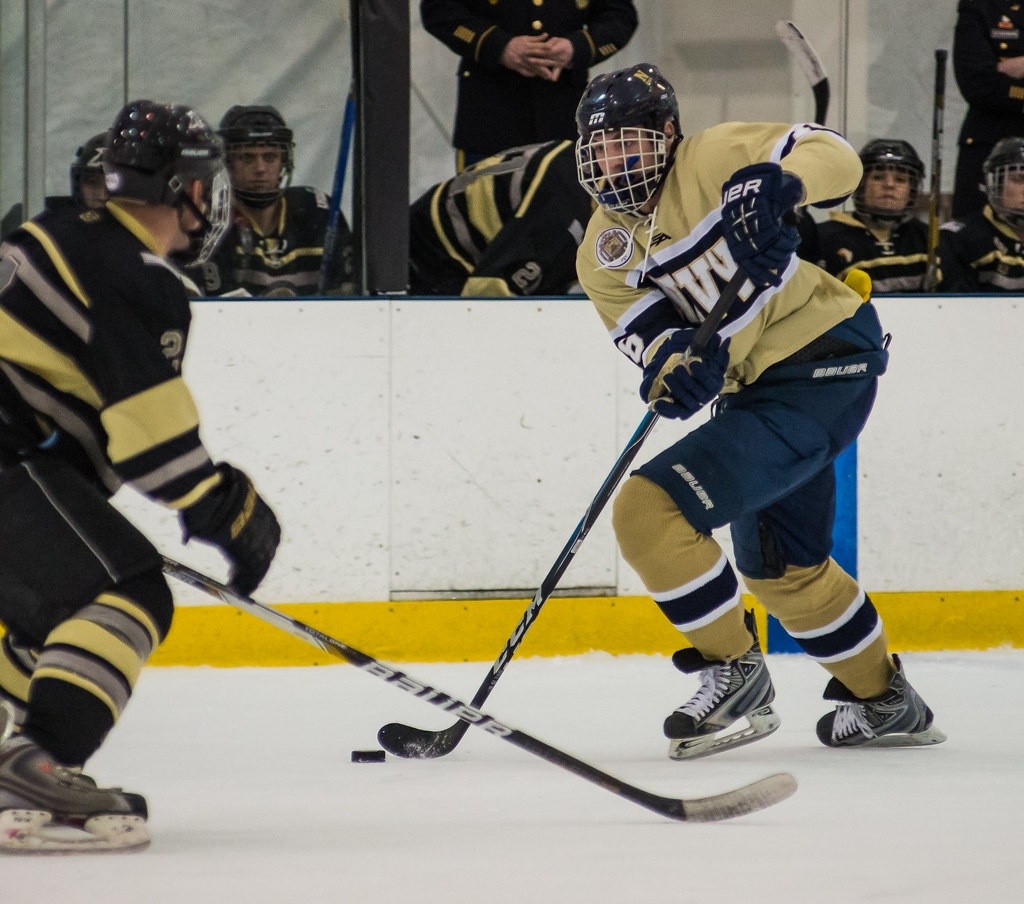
350,750,385,764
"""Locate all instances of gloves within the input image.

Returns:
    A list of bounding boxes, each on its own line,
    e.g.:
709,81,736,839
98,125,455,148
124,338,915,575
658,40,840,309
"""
720,162,807,288
639,328,730,420
194,461,280,597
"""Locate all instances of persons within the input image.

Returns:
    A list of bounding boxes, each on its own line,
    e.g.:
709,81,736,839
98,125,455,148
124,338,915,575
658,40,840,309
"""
416,0,637,171
183,105,359,298
408,135,595,296
573,63,947,758
0,129,114,242
951,0,1024,223
939,135,1024,292
814,137,947,293
0,98,282,853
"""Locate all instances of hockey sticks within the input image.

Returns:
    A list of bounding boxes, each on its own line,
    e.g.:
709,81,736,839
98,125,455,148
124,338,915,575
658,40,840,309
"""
775,19,832,129
375,211,797,760
923,47,949,292
159,551,798,826
314,91,356,297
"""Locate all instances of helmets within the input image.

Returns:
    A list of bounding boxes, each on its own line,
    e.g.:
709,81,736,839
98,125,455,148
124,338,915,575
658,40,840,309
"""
213,107,295,206
97,98,232,267
982,137,1024,226
853,138,926,228
68,130,112,209
576,61,681,217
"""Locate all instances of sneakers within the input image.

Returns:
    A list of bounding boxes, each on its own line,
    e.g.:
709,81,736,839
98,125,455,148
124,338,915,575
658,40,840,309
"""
663,610,783,760
813,652,947,750
0,701,149,854
